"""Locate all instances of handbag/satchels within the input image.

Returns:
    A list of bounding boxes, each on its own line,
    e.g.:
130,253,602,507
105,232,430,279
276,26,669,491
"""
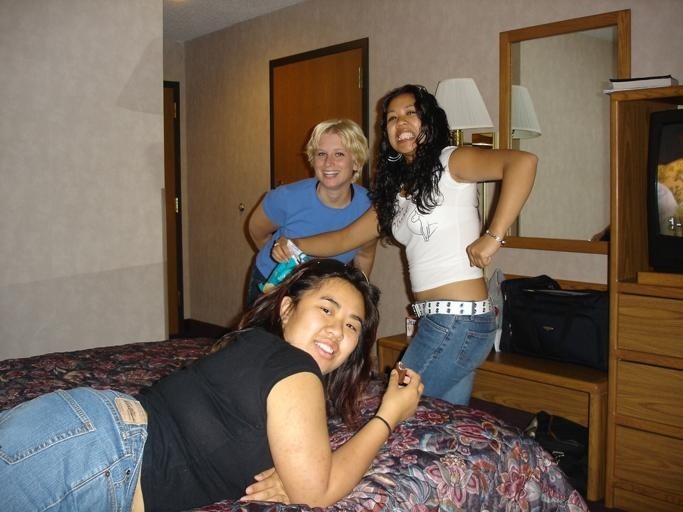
507,285,608,377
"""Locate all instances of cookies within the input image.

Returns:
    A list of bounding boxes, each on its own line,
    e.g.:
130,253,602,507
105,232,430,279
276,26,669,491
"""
396,361,406,383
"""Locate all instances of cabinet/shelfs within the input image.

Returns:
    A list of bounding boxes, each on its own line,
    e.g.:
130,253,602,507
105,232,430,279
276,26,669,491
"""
605,84,683,512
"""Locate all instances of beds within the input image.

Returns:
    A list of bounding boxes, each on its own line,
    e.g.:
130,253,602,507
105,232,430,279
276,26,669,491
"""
2,336,592,512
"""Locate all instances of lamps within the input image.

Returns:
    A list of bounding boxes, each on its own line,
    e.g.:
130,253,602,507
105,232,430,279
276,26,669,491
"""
511,85,542,139
435,76,496,149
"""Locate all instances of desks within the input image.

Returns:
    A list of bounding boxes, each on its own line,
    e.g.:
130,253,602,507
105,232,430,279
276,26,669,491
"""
377,274,608,502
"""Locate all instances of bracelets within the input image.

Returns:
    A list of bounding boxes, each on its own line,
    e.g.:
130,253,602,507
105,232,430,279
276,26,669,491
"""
484,227,509,248
374,412,394,433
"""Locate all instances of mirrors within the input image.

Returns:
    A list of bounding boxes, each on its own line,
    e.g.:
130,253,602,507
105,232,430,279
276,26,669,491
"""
498,9,632,255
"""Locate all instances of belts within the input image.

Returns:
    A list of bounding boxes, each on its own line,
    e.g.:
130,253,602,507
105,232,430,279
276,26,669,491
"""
411,300,493,318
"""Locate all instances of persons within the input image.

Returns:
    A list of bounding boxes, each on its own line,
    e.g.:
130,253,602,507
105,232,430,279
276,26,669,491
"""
244,117,378,307
1,259,425,507
271,82,537,410
